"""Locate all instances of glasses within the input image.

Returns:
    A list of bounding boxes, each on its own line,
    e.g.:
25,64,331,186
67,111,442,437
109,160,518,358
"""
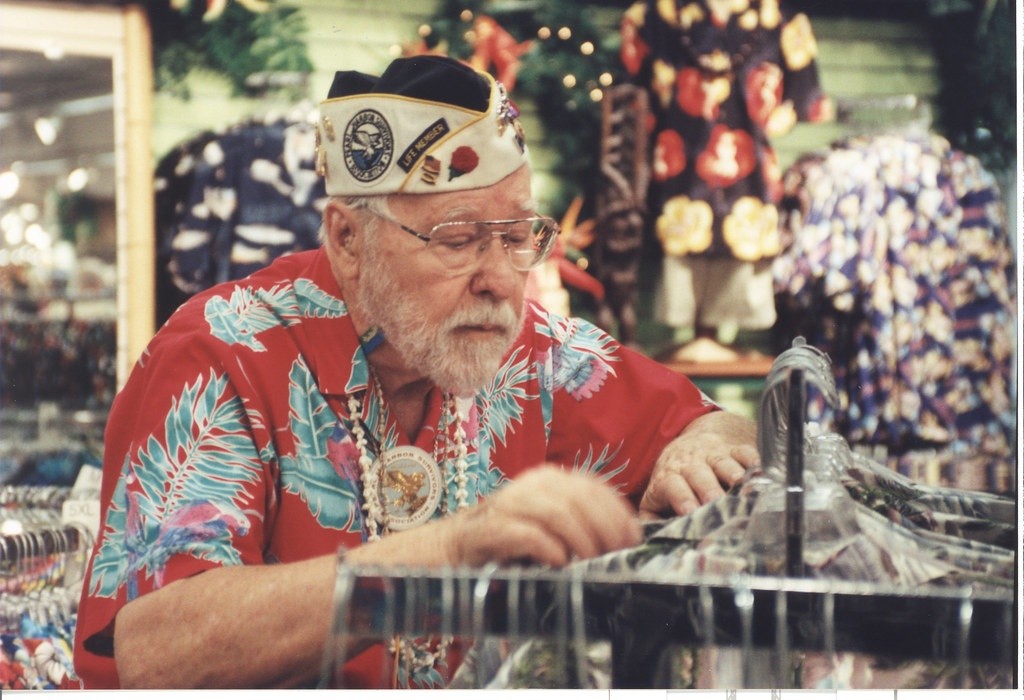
356,202,562,271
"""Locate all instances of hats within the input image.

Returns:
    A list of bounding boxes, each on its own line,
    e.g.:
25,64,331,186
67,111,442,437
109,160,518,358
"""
314,56,528,197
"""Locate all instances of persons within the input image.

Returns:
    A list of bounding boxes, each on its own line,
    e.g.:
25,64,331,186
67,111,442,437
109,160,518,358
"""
72,53,761,690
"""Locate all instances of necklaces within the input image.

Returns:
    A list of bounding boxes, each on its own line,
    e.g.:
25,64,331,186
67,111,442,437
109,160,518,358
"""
346,365,470,673
